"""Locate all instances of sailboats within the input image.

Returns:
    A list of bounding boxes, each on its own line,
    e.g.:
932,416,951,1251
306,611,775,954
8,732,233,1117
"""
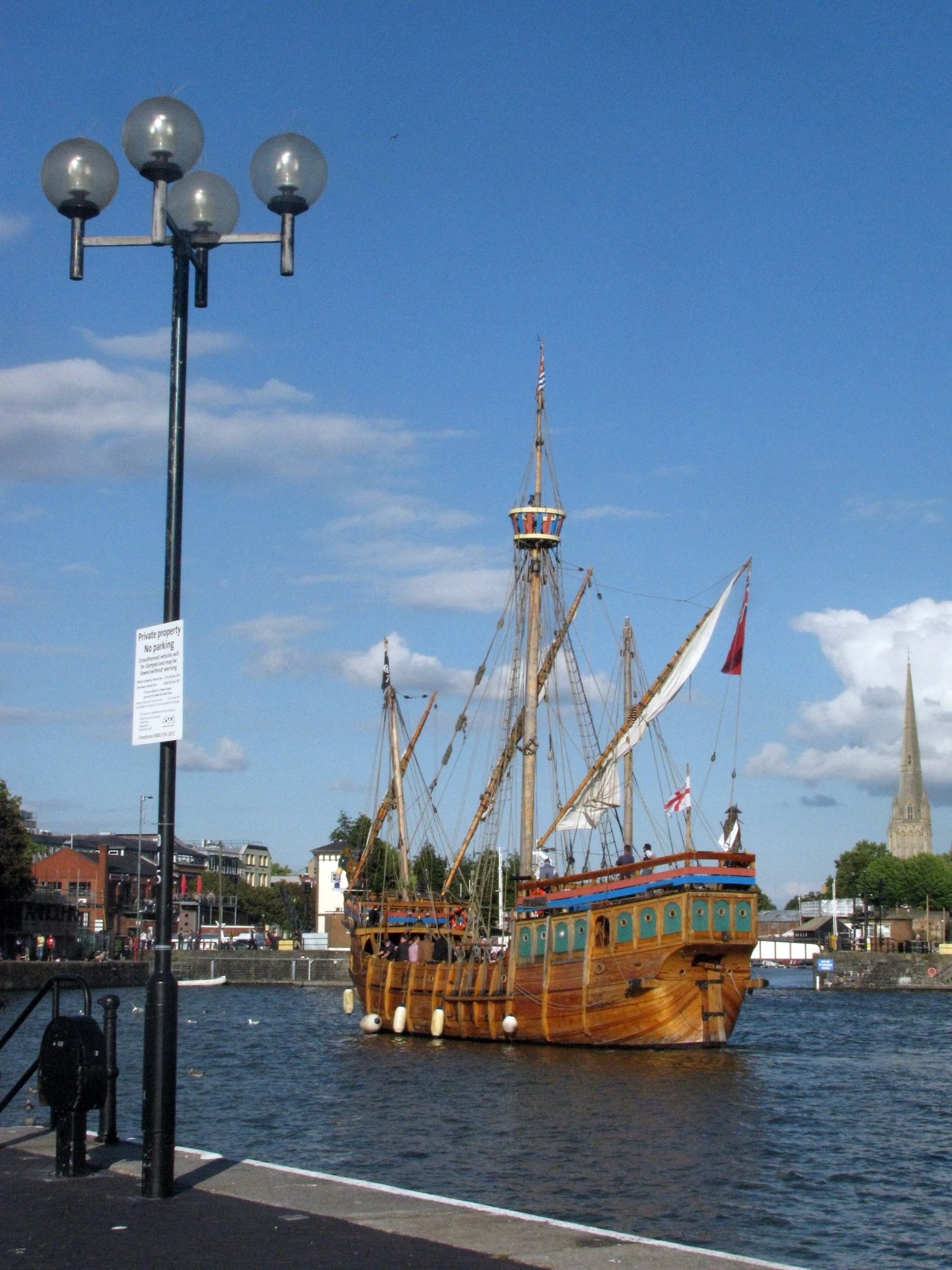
337,335,757,1048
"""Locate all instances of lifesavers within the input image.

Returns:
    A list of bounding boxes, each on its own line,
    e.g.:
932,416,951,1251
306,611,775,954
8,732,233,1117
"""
528,889,547,915
450,907,469,931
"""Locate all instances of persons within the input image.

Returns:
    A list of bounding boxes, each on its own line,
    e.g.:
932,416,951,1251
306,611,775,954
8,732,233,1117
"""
15,933,56,961
267,929,273,950
615,845,634,880
140,930,152,950
364,932,504,963
535,859,557,892
90,949,112,962
293,928,301,949
177,927,201,950
642,843,653,874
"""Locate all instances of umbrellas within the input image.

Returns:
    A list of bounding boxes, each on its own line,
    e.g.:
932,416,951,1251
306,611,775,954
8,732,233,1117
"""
182,874,202,896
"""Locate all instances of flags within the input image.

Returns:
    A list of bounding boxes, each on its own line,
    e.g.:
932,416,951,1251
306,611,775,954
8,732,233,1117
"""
663,776,692,816
721,573,749,676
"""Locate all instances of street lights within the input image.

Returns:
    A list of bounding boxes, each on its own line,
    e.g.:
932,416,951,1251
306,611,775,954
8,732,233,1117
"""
136,795,153,961
41,95,325,1200
205,842,222,951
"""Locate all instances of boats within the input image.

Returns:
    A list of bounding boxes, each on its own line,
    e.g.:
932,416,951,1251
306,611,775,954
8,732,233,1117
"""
157,975,226,986
